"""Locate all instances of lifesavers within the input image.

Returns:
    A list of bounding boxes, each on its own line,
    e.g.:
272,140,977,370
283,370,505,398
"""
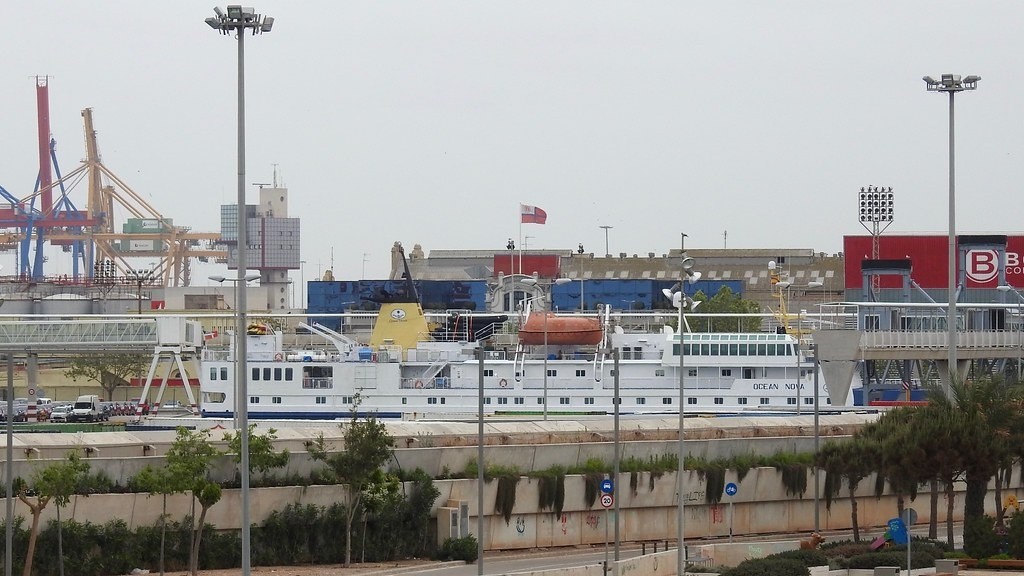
498,377,508,388
413,379,425,390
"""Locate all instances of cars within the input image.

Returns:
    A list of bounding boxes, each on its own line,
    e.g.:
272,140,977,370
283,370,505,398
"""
0,398,74,422
100,401,192,422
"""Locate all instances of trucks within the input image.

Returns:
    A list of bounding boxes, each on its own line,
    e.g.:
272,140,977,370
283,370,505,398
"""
71,394,99,423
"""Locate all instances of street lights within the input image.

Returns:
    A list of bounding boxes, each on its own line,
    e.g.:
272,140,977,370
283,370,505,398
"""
681,232,687,250
921,72,982,411
521,276,572,421
207,272,263,428
661,249,705,576
775,281,823,415
577,242,585,314
597,305,625,565
506,238,515,314
205,4,276,576
599,225,613,255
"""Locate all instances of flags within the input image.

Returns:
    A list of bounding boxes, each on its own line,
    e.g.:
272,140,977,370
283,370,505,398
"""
520,204,547,224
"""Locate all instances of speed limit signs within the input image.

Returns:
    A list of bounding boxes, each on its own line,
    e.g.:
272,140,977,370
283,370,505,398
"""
600,493,614,509
